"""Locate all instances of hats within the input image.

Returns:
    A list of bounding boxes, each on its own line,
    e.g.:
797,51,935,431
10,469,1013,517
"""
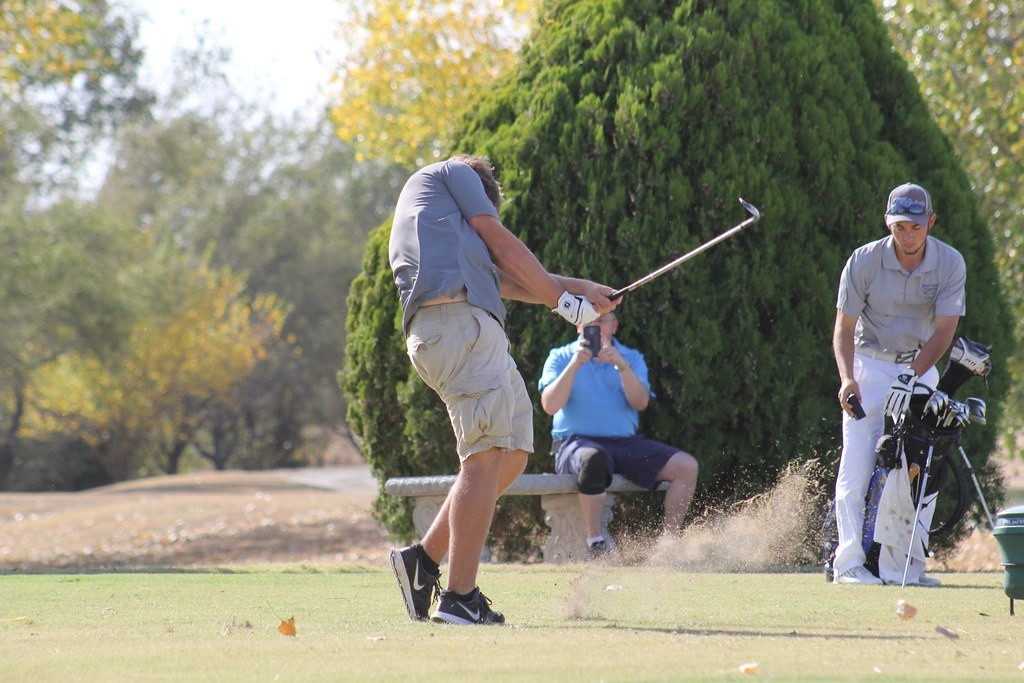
887,182,932,225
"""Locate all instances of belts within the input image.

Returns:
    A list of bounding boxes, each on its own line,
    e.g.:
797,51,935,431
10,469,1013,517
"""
855,346,920,363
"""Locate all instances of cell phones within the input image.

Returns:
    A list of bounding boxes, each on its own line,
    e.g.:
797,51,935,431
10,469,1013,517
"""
584,325,601,356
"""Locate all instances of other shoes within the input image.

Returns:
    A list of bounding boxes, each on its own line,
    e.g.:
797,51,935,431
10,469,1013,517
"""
586,541,607,561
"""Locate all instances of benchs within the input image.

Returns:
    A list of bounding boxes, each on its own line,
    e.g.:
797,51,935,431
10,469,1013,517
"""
386,474,667,576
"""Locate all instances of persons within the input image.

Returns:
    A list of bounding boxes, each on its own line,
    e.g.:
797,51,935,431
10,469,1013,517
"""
833,182,966,586
537,306,698,561
388,153,622,626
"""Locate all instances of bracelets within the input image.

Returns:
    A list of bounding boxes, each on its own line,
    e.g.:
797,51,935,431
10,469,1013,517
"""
618,363,630,371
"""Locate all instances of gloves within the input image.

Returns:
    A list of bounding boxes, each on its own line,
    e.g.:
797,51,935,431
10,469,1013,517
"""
882,368,919,416
552,290,602,325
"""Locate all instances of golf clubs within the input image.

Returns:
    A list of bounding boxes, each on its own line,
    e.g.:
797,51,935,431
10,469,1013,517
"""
919,388,986,431
607,195,760,302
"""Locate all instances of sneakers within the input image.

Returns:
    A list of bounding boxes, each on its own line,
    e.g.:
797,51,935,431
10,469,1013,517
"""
834,566,883,586
389,544,443,622
918,573,940,586
430,585,505,625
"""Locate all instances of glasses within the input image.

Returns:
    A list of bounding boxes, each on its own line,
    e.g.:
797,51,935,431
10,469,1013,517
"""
887,204,927,215
